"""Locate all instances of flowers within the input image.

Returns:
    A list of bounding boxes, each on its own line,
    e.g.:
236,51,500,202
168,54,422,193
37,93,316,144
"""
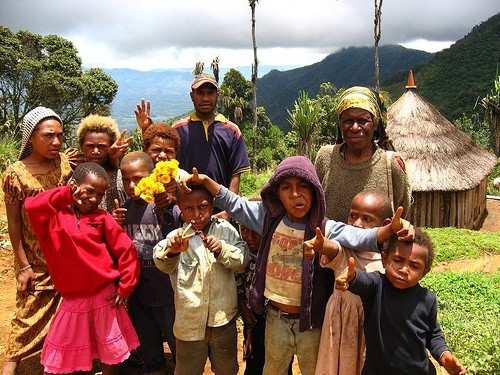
134,159,180,204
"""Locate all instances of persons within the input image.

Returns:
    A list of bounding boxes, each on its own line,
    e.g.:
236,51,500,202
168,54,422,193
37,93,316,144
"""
335,227,467,375
314,86,413,225
75,74,251,375
153,183,250,375
234,198,294,375
26,164,139,375
304,189,392,374
0,107,86,375
182,157,414,375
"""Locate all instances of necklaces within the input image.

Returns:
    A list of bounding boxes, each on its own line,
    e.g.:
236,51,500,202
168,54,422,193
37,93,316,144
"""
75,209,82,234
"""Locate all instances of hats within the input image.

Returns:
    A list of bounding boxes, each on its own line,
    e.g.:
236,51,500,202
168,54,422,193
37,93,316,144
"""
191,73,219,92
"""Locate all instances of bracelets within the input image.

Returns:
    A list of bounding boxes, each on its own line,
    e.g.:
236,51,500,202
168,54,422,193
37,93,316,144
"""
16,265,32,272
